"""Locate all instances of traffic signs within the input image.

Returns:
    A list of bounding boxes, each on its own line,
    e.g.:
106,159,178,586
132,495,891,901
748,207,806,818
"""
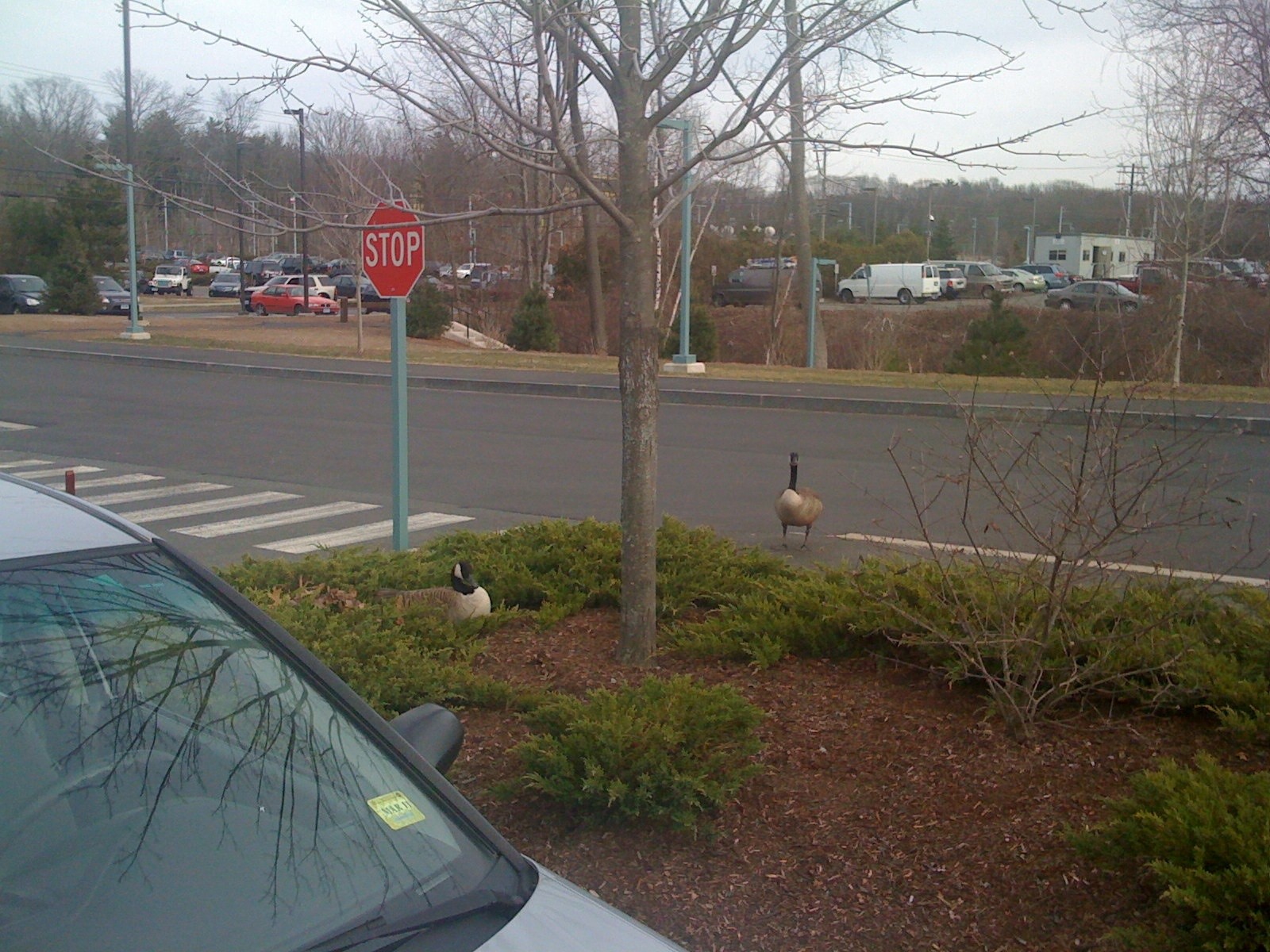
360,199,426,300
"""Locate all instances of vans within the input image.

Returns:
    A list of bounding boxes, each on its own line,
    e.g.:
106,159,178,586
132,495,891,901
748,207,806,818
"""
922,259,1015,299
710,255,824,311
938,266,968,301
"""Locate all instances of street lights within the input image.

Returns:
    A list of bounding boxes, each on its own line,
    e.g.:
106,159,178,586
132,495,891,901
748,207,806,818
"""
284,108,309,313
838,202,852,230
234,140,253,316
861,187,878,246
1022,196,1037,265
926,182,941,261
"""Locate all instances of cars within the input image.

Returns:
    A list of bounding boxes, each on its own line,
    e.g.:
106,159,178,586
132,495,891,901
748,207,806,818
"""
143,246,522,318
208,272,242,299
1,468,687,952
1012,263,1071,289
1000,267,1046,294
1101,256,1270,299
1043,280,1151,315
249,283,340,316
0,273,53,315
91,275,139,317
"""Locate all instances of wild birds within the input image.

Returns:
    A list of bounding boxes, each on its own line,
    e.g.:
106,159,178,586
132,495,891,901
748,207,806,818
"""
450,561,479,596
775,451,824,551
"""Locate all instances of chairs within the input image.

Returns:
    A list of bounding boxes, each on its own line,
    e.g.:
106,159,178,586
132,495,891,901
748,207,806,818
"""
0,692,79,882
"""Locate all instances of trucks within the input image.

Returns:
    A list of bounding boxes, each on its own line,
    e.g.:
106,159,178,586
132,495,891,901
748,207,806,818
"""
836,263,942,305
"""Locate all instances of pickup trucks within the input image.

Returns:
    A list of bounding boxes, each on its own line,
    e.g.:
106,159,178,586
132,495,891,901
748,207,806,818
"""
148,264,193,297
